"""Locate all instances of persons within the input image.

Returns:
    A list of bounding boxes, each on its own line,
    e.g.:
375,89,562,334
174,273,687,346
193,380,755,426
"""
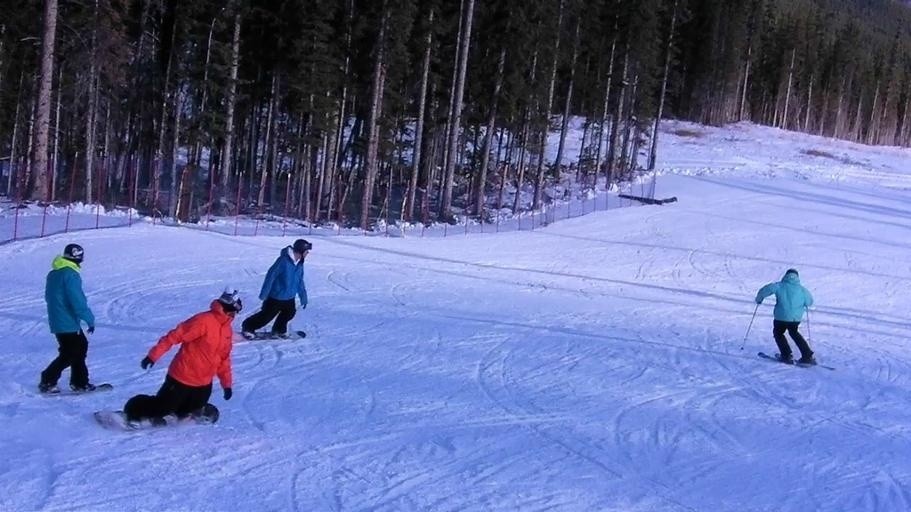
239,238,314,340
122,287,244,417
36,243,116,398
756,269,815,363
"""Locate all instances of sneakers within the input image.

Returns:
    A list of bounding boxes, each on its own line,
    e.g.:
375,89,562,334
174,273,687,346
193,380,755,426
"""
241,329,257,339
70,382,96,392
39,382,60,394
270,331,293,339
776,353,813,363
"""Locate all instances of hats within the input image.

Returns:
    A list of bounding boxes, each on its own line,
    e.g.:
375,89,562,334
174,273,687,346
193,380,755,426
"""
62,243,85,263
218,285,242,315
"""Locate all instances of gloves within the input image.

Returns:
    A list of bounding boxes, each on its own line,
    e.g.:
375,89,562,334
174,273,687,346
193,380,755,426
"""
140,355,154,369
222,387,232,400
86,325,94,335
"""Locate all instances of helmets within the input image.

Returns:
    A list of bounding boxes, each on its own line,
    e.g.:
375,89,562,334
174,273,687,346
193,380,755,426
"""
293,238,313,254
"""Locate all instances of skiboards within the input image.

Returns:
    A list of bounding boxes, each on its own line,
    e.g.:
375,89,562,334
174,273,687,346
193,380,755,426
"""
757,351,834,371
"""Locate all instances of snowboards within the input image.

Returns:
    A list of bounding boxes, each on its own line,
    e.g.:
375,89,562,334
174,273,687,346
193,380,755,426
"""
95,402,219,432
230,331,307,345
25,383,114,399
